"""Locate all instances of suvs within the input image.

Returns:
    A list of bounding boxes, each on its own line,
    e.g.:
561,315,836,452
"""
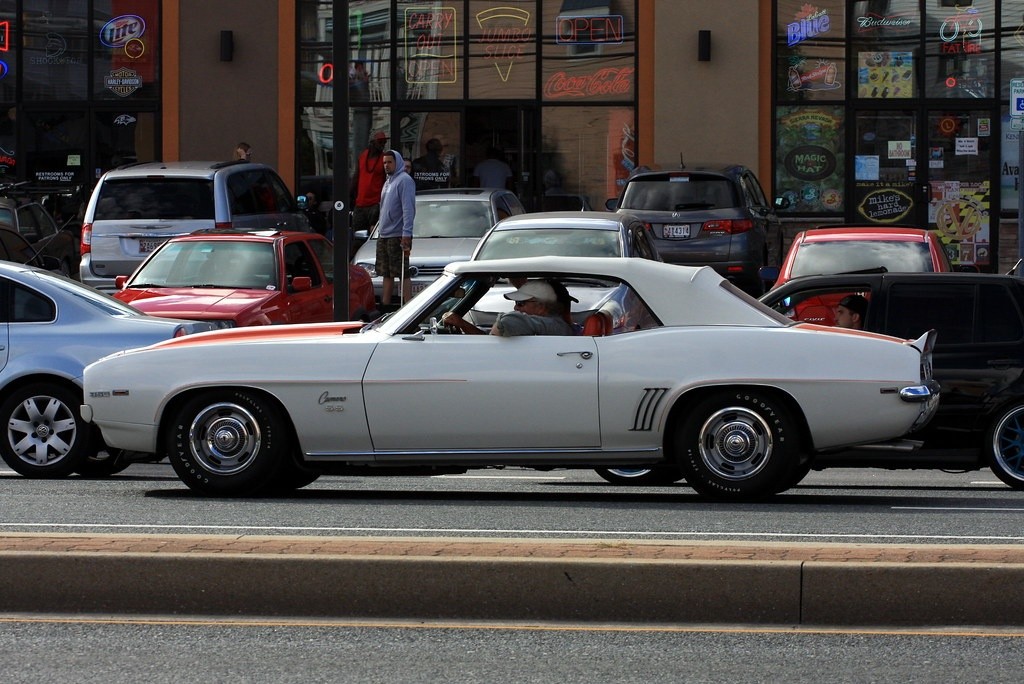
75,157,325,296
606,162,792,299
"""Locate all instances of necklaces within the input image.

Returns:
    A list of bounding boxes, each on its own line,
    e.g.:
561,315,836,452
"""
366,149,380,173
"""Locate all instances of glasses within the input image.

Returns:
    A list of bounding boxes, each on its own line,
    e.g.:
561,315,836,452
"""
516,300,537,307
378,140,387,144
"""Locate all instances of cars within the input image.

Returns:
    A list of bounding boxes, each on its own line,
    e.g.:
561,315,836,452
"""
0,258,220,481
758,225,983,327
110,226,379,327
1,182,88,282
461,208,664,330
350,185,528,303
591,265,1024,494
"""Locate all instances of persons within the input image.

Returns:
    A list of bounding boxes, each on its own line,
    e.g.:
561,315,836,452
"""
834,295,868,329
473,150,514,192
350,130,387,262
404,139,449,191
374,150,416,314
442,278,582,335
307,192,327,235
234,143,252,161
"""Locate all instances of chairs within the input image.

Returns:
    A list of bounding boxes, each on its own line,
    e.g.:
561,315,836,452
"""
578,308,613,336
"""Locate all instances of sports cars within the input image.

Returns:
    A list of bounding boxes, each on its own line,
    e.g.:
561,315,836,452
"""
80,252,944,506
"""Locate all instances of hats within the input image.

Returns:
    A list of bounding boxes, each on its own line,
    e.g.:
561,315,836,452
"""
370,132,385,142
546,279,579,304
503,279,556,303
841,296,869,318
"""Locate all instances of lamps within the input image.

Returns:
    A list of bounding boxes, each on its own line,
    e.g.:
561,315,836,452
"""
218,29,235,63
697,30,712,62
